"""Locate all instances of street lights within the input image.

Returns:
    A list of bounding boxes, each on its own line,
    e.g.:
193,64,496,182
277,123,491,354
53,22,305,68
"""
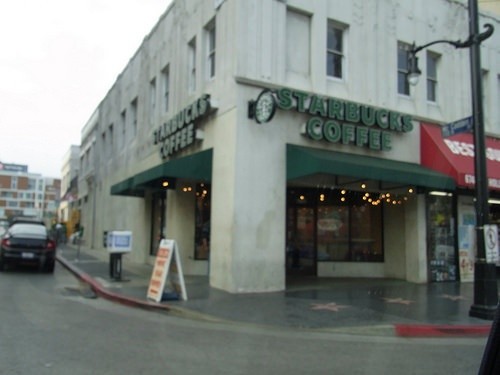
406,22,500,319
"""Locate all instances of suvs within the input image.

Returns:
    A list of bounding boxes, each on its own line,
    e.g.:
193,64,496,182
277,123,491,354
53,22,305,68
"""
1,219,56,274
